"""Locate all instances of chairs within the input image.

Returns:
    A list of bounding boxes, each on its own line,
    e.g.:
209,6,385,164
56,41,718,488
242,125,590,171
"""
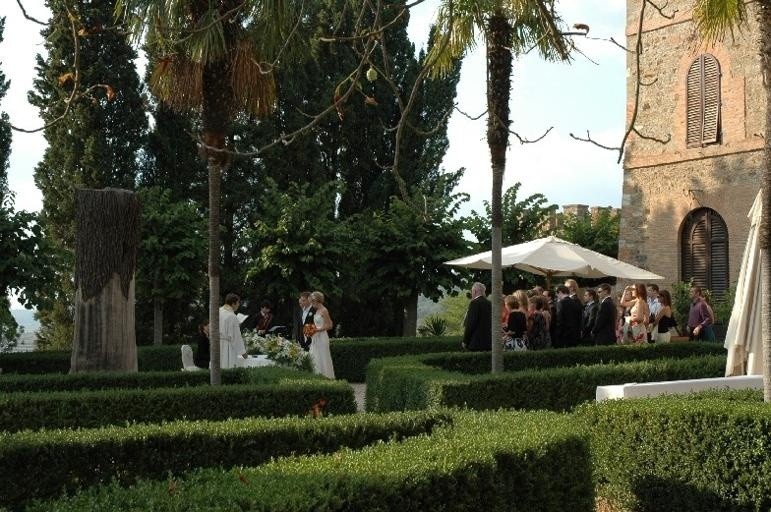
178,340,201,374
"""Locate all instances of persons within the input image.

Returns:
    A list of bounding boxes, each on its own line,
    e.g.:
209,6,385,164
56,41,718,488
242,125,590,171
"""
299,290,317,351
242,298,274,330
307,290,336,381
218,294,248,371
460,278,716,352
195,317,209,371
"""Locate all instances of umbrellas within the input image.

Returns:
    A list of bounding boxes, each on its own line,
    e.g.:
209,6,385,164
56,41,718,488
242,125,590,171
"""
722,183,770,402
441,231,666,294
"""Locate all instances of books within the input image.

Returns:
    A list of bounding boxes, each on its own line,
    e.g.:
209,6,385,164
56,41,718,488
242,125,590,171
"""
234,311,248,325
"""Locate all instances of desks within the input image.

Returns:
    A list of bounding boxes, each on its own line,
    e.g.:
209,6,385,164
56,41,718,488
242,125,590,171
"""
232,351,278,368
